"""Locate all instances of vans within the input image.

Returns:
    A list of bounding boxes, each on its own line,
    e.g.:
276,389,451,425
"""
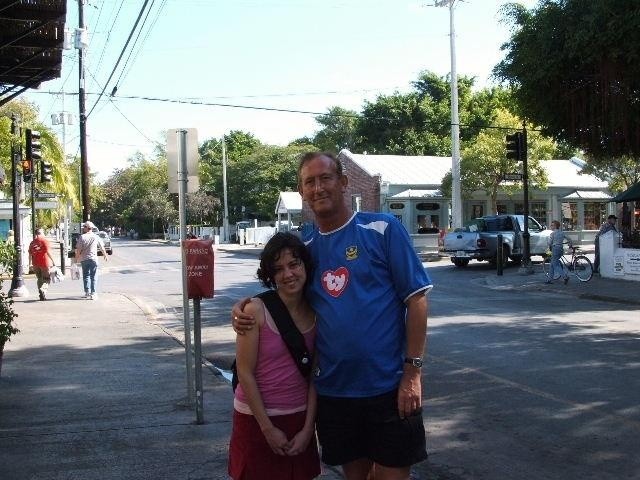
266,220,294,234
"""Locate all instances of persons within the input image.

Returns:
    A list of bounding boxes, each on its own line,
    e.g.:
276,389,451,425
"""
3,229,15,274
228,232,322,480
231,152,434,480
592,215,618,273
28,228,55,301
75,221,108,300
543,220,572,285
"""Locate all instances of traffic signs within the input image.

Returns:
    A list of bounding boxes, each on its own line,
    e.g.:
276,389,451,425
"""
33,193,55,199
34,202,58,209
504,173,522,180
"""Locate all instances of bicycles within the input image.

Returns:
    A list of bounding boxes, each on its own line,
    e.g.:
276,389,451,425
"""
542,245,594,284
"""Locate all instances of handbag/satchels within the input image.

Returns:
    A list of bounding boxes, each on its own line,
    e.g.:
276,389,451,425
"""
51,266,63,283
70,268,80,280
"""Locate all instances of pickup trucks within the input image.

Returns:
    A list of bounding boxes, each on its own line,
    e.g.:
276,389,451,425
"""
439,215,563,269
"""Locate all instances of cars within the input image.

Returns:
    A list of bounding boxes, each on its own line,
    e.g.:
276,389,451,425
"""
70,222,99,237
54,223,70,236
94,231,113,256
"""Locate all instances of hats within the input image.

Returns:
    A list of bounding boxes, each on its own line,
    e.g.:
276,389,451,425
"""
84,221,92,229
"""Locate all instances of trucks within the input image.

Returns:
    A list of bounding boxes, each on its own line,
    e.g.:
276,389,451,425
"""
229,220,253,243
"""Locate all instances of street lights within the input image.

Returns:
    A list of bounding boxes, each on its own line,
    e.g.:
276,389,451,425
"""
8,113,31,296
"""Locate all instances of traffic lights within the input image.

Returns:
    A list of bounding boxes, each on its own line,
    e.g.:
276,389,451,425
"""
22,160,32,184
40,161,52,183
25,129,42,160
506,132,523,164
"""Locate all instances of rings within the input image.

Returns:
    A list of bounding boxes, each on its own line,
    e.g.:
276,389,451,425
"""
234,316,238,319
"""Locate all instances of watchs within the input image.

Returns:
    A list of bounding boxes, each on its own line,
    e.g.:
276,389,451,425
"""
404,356,423,368
